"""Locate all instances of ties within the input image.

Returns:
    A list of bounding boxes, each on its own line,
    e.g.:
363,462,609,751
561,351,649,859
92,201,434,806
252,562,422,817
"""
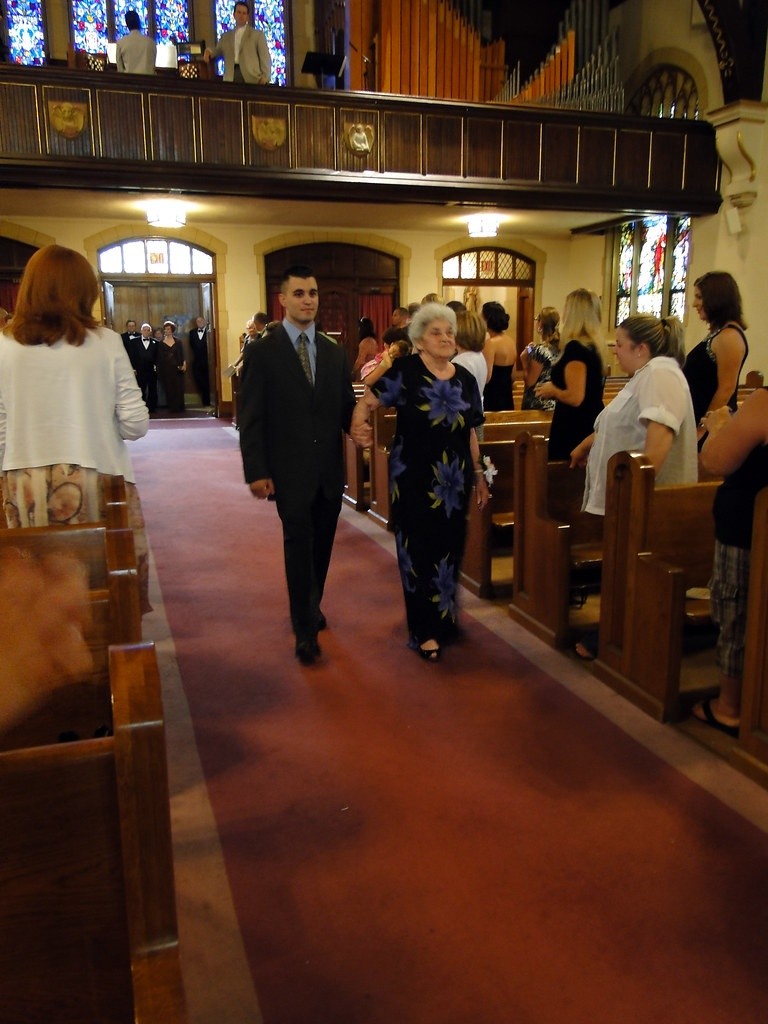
298,333,313,388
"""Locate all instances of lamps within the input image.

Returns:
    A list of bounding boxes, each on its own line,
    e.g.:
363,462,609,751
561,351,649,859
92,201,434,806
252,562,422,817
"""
177,40,206,55
301,52,345,88
145,208,187,229
466,220,500,238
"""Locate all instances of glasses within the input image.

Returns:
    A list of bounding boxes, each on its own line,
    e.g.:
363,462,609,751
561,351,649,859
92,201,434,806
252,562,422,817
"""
533,315,541,322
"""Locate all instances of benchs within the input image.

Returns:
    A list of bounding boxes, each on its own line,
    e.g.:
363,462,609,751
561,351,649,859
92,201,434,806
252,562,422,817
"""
0,473,190,1024
340,372,768,787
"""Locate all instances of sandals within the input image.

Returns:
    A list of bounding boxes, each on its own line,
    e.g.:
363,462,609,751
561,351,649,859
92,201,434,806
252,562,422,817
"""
417,641,442,661
573,635,599,660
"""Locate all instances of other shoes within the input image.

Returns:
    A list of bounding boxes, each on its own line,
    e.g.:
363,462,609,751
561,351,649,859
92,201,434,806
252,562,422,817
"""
295,639,320,664
317,608,327,629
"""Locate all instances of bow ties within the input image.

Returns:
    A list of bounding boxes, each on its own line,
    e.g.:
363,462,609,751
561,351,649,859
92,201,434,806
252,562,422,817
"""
143,338,149,341
128,333,136,336
198,329,203,332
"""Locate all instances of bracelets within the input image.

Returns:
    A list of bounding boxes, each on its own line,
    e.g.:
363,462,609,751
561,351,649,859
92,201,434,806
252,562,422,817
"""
474,469,484,473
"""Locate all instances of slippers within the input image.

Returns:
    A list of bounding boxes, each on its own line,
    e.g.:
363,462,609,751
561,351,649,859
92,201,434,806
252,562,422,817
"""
690,697,740,736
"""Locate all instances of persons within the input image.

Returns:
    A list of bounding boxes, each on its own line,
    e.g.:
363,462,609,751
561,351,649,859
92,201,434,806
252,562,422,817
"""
482,301,518,410
128,323,156,418
534,288,607,626
189,318,217,406
230,275,560,414
116,11,157,75
699,385,768,727
681,271,751,434
570,313,700,658
239,268,371,670
203,2,272,84
0,245,149,529
120,320,141,355
353,303,492,665
154,321,187,418
